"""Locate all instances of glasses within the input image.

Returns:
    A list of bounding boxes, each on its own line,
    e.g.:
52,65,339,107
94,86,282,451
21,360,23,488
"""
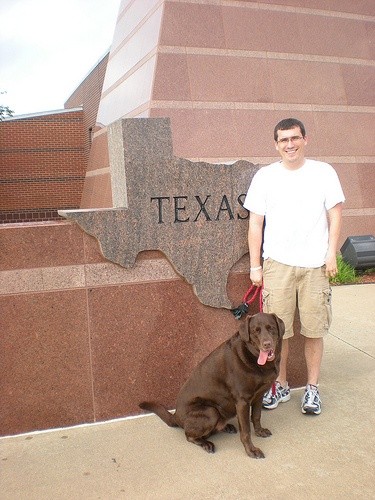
277,135,303,144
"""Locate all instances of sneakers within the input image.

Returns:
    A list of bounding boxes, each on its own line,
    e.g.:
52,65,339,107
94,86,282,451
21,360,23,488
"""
262,380,291,410
300,383,322,415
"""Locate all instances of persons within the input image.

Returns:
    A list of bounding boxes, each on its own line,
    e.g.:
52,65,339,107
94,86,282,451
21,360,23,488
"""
243,118,346,414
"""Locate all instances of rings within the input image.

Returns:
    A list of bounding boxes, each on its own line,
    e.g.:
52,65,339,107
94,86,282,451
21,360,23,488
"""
253,283,255,285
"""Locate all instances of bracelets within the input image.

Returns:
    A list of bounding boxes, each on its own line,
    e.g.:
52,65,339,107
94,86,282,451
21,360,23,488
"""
250,266,262,271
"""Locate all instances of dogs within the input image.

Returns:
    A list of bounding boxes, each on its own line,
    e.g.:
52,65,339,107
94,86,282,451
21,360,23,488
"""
138,313,285,460
